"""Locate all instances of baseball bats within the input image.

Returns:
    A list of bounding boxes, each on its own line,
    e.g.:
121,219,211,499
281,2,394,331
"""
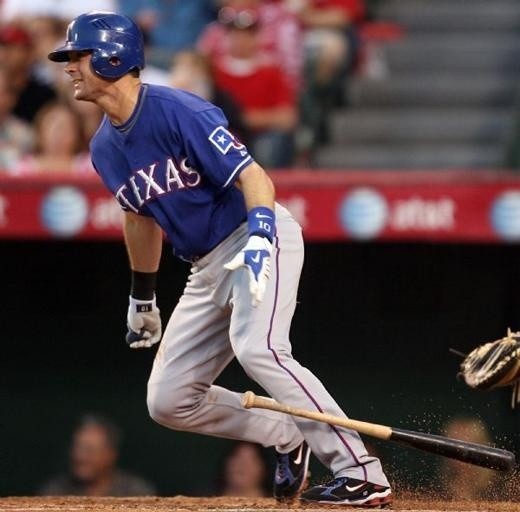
242,390,515,472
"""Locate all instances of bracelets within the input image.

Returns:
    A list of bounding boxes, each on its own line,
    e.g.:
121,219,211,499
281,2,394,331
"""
247,206,275,244
131,270,159,301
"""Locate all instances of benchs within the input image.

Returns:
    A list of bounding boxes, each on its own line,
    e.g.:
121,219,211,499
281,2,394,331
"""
310,0,519,168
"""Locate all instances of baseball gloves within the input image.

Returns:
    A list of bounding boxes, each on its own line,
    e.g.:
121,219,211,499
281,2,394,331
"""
456,331,520,409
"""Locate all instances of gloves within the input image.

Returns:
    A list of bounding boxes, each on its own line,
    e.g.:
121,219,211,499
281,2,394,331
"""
125,293,162,349
224,235,272,308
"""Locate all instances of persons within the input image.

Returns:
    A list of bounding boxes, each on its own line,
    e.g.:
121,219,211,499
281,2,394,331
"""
438,415,520,503
217,439,271,497
38,411,158,496
0,0,359,169
48,11,392,509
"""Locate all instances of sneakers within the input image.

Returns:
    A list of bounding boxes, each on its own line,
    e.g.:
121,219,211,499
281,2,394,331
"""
272,441,311,499
299,477,391,505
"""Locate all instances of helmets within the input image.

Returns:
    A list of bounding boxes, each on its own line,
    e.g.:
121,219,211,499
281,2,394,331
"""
48,10,146,79
